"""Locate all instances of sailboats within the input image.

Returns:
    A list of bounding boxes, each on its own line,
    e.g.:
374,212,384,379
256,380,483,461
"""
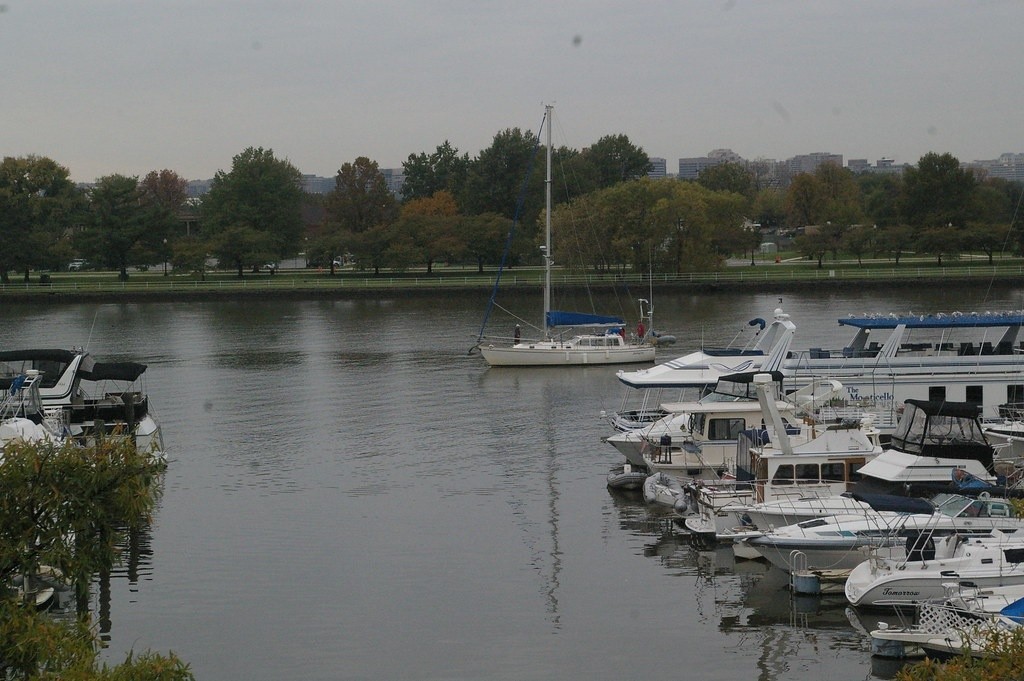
471,106,657,366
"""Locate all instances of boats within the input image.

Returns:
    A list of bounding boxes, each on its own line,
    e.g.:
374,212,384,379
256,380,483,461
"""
605,487,907,681
598,307,1023,659
1,345,168,614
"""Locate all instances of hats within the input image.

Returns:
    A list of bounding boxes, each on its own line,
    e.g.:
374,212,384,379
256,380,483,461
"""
637,320,641,322
516,324,520,327
620,327,622,329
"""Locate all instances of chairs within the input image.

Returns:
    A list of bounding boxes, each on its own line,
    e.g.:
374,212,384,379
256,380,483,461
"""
843,342,1015,358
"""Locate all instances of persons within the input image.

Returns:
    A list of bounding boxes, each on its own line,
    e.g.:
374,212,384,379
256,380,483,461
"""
637,320,644,345
514,324,521,345
